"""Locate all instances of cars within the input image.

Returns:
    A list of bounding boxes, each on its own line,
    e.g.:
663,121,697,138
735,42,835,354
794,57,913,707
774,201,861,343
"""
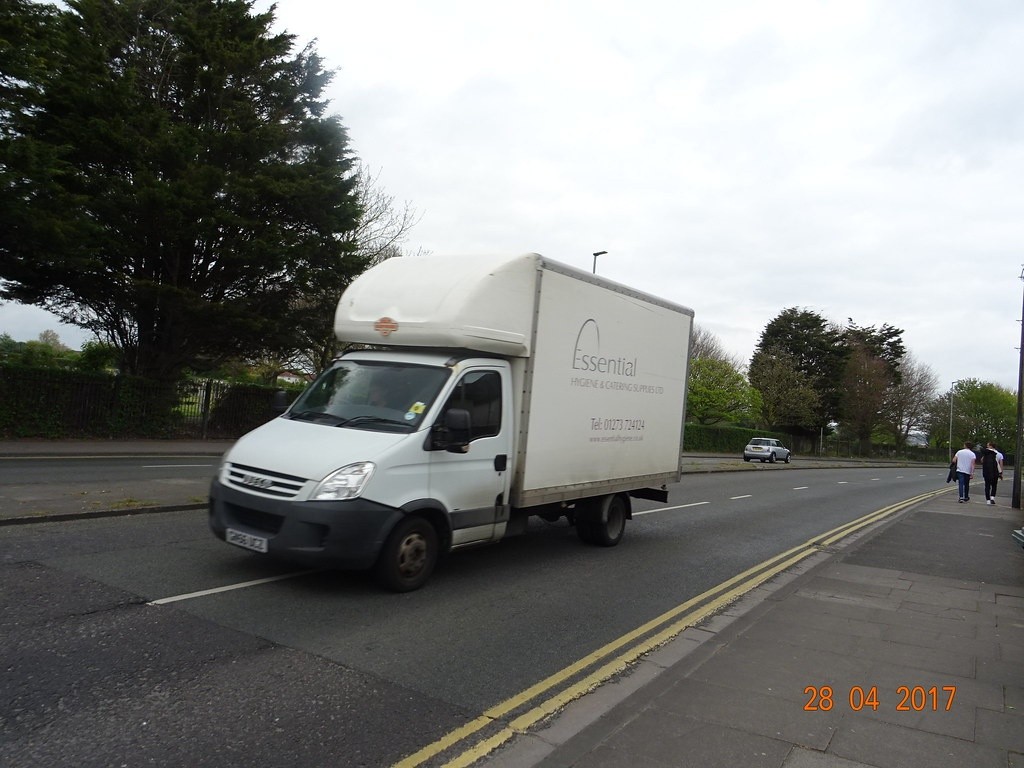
744,437,791,463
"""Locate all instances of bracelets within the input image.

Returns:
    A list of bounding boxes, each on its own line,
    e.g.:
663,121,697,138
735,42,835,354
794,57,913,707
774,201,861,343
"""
999,472,1002,474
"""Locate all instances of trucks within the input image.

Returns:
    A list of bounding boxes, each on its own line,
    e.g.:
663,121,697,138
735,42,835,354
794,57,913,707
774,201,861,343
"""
204,252,695,599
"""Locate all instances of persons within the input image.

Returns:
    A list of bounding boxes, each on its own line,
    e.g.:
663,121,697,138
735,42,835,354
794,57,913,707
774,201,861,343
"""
980,441,1004,505
952,441,976,503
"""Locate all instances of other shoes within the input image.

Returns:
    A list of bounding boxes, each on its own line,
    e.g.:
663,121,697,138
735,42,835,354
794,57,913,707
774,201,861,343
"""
991,497,996,504
958,498,964,503
987,500,991,504
964,497,969,501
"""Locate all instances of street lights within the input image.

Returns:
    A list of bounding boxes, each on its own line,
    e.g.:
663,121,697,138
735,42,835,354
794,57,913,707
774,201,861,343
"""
949,381,959,465
590,250,609,273
